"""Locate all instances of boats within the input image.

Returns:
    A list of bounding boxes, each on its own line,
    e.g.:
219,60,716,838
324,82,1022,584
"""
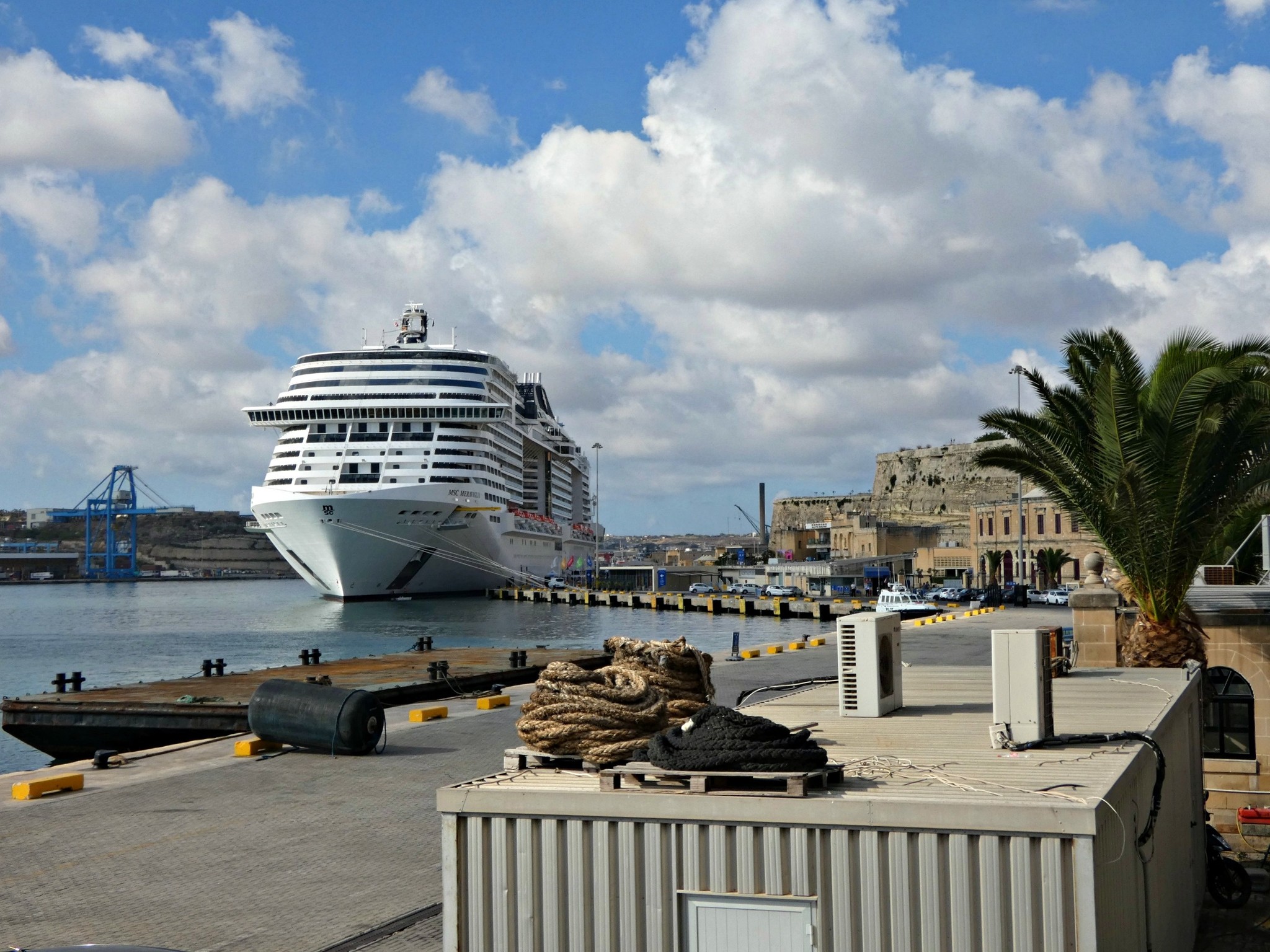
844,559,944,621
573,523,594,535
507,507,557,526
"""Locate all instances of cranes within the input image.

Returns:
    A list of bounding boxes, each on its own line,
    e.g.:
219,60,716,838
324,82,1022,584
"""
45,465,196,579
733,504,771,542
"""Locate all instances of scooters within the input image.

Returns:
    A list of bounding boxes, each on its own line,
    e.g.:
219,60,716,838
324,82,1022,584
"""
1203,790,1253,910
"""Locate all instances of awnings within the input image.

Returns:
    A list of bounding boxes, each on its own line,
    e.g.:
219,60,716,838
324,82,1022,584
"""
864,566,890,577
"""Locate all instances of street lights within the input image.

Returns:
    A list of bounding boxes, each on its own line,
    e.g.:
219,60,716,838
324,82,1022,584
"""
199,526,205,572
1008,367,1029,585
591,443,604,591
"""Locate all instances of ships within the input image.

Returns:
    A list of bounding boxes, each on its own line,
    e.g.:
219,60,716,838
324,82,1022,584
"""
240,299,604,604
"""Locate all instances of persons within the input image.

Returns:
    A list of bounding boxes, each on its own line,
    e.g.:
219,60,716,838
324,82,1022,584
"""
865,585,870,597
850,581,856,597
922,581,930,591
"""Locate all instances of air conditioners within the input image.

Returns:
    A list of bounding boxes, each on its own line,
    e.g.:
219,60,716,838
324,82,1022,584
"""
989,627,1054,748
1196,565,1234,585
835,610,903,718
1036,626,1063,679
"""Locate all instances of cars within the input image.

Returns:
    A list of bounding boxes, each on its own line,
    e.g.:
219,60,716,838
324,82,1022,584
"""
726,582,744,594
760,584,785,596
689,582,715,593
782,585,803,597
739,583,762,594
913,583,1080,606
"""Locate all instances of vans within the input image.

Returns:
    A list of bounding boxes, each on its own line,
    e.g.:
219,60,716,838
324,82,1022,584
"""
548,578,564,589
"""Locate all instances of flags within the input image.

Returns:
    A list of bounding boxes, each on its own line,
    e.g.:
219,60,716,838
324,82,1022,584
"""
586,556,592,566
560,557,567,570
567,555,574,567
576,556,583,568
551,556,558,569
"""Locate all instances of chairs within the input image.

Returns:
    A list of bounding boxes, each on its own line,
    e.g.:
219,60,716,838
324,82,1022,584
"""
856,590,862,596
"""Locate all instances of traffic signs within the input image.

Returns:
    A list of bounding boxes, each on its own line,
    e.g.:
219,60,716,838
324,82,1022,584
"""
585,569,592,589
657,567,667,589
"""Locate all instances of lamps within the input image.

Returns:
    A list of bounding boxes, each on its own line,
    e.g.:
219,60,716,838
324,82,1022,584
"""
1181,659,1202,681
